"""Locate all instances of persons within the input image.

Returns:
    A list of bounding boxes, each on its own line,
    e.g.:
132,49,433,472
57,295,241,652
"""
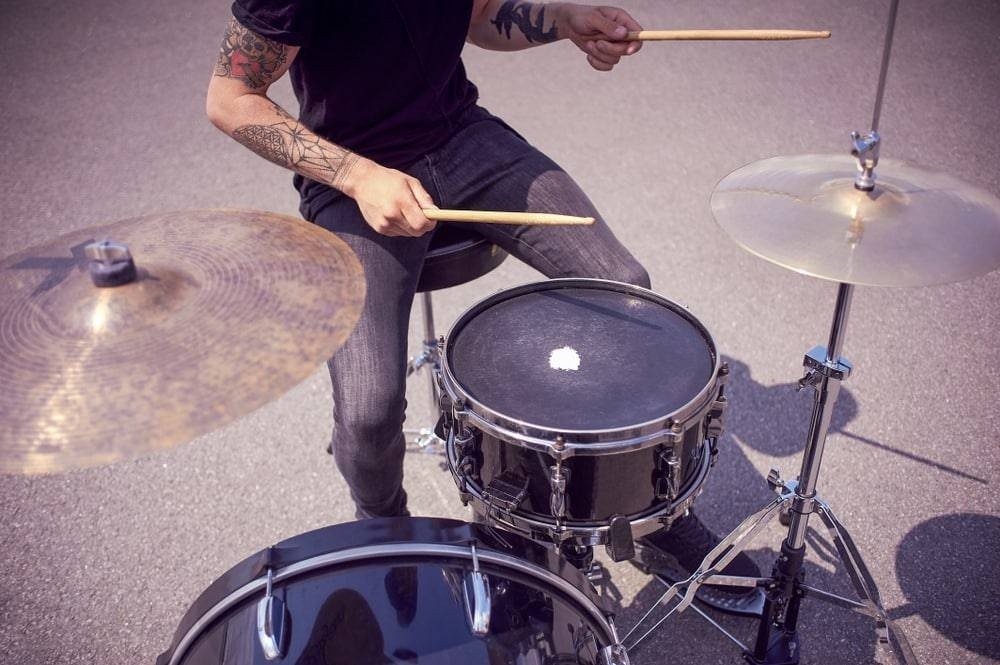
205,0,762,597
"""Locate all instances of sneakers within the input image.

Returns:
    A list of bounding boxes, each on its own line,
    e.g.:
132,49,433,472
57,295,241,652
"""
632,509,761,599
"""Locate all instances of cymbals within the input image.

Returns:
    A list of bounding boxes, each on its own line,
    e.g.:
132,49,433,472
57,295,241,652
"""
0,205,369,476
708,150,1000,289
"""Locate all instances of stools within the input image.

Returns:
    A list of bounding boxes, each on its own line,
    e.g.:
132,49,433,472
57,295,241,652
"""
327,223,508,459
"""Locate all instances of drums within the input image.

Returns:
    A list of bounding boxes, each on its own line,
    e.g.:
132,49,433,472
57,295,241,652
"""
155,515,632,665
433,277,728,563
